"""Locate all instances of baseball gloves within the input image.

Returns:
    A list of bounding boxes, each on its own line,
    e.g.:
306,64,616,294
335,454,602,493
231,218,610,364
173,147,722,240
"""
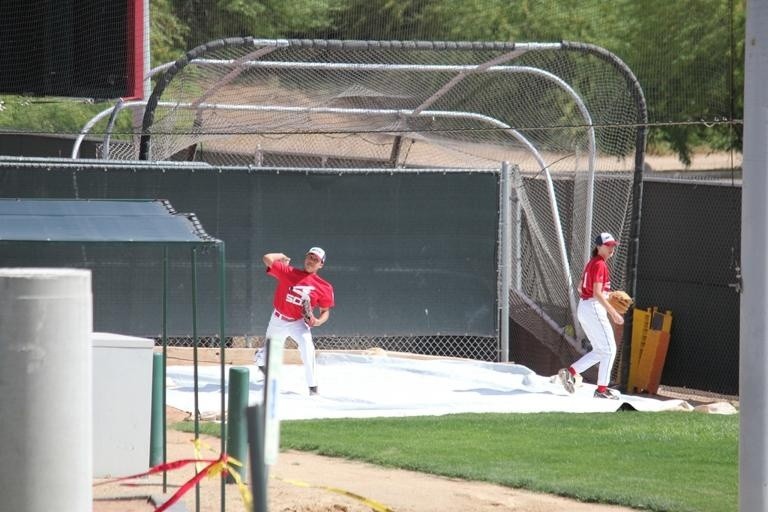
302,300,315,328
609,291,632,314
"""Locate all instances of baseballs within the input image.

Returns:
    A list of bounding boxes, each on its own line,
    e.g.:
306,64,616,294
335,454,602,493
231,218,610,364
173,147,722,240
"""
285,260,290,265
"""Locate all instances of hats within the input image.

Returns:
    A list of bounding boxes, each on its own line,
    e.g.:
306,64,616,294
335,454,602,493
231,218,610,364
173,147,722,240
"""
305,246,326,264
595,233,619,246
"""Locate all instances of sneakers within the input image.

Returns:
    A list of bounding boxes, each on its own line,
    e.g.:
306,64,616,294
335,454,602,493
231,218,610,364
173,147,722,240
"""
593,389,621,400
308,384,319,396
558,367,577,394
253,349,266,374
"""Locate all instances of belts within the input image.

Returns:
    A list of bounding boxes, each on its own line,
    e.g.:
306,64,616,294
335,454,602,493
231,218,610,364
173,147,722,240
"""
274,311,305,323
582,296,589,301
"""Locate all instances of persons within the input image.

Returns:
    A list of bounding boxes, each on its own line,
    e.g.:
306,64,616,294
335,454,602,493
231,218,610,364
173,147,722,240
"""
253,243,334,396
553,227,629,404
